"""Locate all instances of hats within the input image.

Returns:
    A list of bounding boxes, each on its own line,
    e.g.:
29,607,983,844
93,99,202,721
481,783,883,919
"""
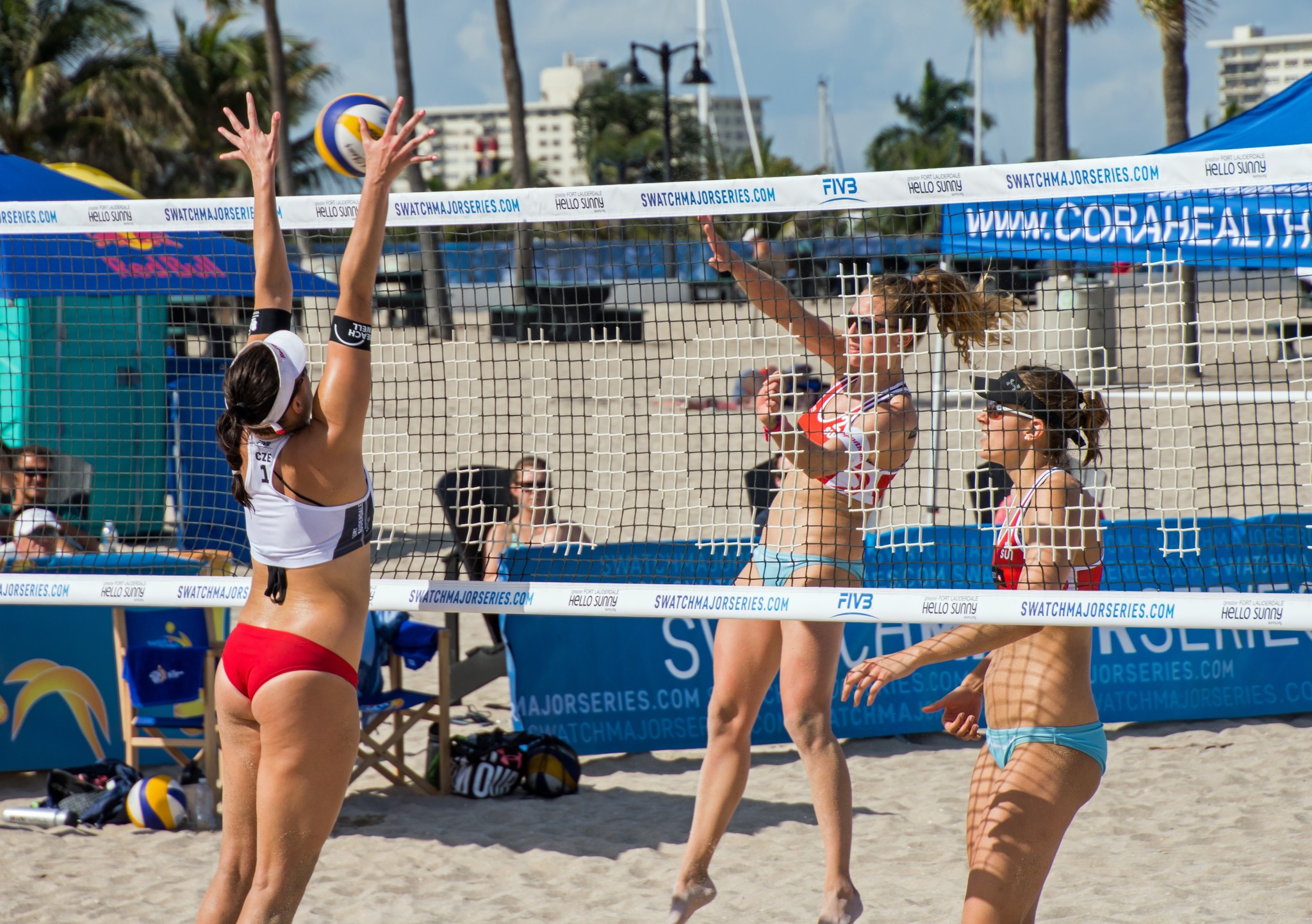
13,508,61,537
970,369,1086,449
223,330,308,434
788,363,812,378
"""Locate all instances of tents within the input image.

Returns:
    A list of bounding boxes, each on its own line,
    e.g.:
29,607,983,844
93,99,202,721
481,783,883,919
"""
917,70,1312,521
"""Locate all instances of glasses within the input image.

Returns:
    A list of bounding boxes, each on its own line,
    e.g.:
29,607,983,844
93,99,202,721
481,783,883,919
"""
847,311,905,335
283,366,307,416
777,472,788,479
31,528,55,536
987,401,1037,420
16,467,54,477
516,480,551,490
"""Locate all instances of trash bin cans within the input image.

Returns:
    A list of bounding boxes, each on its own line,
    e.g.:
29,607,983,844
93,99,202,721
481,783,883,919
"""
1036,278,1119,386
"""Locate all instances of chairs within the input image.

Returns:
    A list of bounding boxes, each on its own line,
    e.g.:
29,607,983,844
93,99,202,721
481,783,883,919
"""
433,463,518,660
108,549,454,802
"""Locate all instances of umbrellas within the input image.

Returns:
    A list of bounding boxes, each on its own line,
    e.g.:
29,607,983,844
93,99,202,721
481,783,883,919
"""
1,158,339,565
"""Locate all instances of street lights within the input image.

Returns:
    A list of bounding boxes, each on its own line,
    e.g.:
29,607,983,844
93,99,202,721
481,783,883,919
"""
619,37,714,286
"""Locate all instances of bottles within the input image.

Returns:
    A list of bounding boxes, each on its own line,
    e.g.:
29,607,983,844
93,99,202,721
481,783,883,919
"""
425,721,440,790
102,519,118,553
194,779,216,831
2,806,82,829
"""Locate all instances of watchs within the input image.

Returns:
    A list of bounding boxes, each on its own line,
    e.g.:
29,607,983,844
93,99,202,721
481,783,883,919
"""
762,413,788,442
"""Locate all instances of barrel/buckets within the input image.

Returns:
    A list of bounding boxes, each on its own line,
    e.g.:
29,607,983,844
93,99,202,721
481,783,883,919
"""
1037,281,1118,385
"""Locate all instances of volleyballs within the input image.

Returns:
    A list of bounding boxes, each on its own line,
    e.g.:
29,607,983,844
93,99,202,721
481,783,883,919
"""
527,753,576,796
314,92,392,177
126,774,188,830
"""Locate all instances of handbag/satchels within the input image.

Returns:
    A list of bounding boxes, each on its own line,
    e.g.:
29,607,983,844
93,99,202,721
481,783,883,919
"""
47,759,144,830
429,728,580,799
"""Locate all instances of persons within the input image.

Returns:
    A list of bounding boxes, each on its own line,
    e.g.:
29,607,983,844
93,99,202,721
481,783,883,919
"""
753,447,790,539
13,509,61,560
994,494,1018,524
199,91,438,924
666,222,1029,924
0,444,99,554
839,366,1109,924
482,455,594,584
